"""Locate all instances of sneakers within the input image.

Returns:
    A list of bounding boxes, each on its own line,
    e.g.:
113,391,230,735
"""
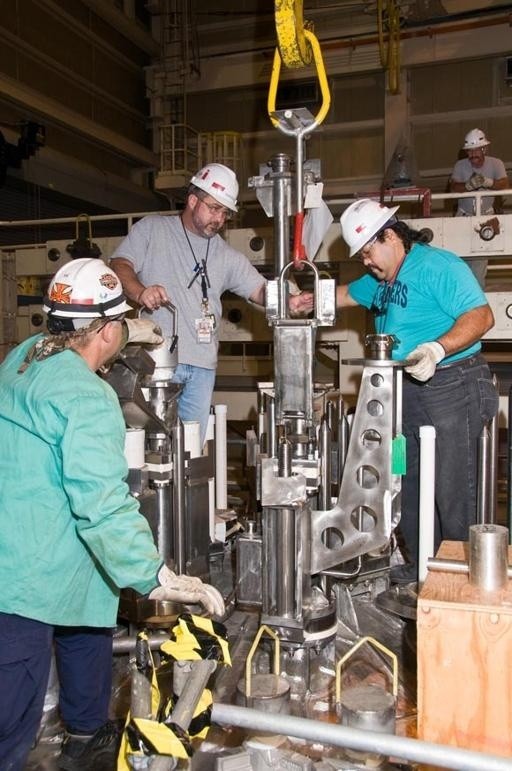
57,720,128,770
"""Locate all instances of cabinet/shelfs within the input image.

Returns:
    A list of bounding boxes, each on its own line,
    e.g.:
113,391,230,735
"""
415,540,512,770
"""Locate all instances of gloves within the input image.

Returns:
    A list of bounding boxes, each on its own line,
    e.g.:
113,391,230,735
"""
122,318,164,352
403,340,446,384
146,560,227,616
465,168,496,192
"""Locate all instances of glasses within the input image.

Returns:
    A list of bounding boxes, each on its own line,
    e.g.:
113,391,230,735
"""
188,192,234,221
349,231,385,262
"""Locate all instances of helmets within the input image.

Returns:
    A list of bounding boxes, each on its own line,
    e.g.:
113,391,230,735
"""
460,129,491,151
189,163,241,214
340,197,400,260
43,257,135,319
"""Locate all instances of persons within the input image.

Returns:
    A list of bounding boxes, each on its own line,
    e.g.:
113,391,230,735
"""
108,163,313,453
450,128,510,217
0,256,226,771
275,198,500,583
